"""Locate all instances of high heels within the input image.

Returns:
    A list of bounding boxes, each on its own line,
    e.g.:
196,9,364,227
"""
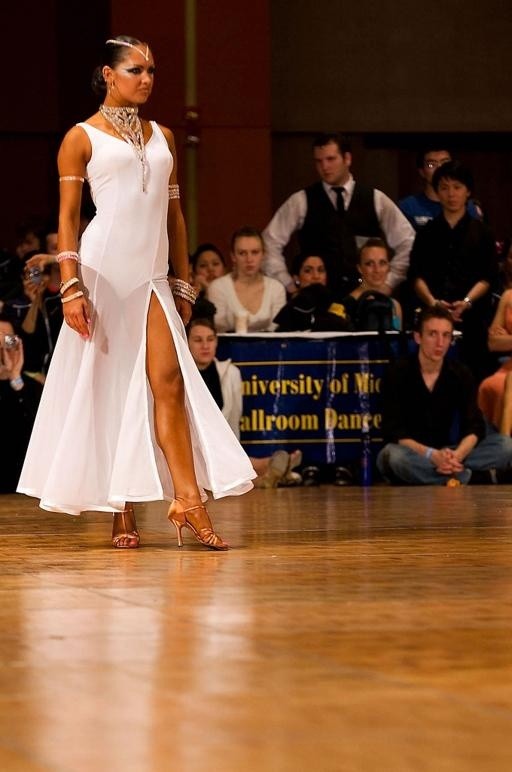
168,499,228,551
112,509,140,548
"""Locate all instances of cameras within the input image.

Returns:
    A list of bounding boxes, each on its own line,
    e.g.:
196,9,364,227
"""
5,334,20,347
29,266,40,282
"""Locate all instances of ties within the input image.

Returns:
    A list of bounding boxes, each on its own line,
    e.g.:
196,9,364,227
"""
332,187,344,211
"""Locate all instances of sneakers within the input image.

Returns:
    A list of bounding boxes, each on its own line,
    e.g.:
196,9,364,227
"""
264,450,290,488
303,466,321,486
335,465,374,486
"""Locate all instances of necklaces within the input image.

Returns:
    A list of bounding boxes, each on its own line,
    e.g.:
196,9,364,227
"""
99,105,151,195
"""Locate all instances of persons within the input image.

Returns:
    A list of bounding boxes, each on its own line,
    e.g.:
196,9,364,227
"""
16,32,257,552
262,135,415,298
396,142,485,228
0,203,96,491
186,228,512,490
408,161,500,338
